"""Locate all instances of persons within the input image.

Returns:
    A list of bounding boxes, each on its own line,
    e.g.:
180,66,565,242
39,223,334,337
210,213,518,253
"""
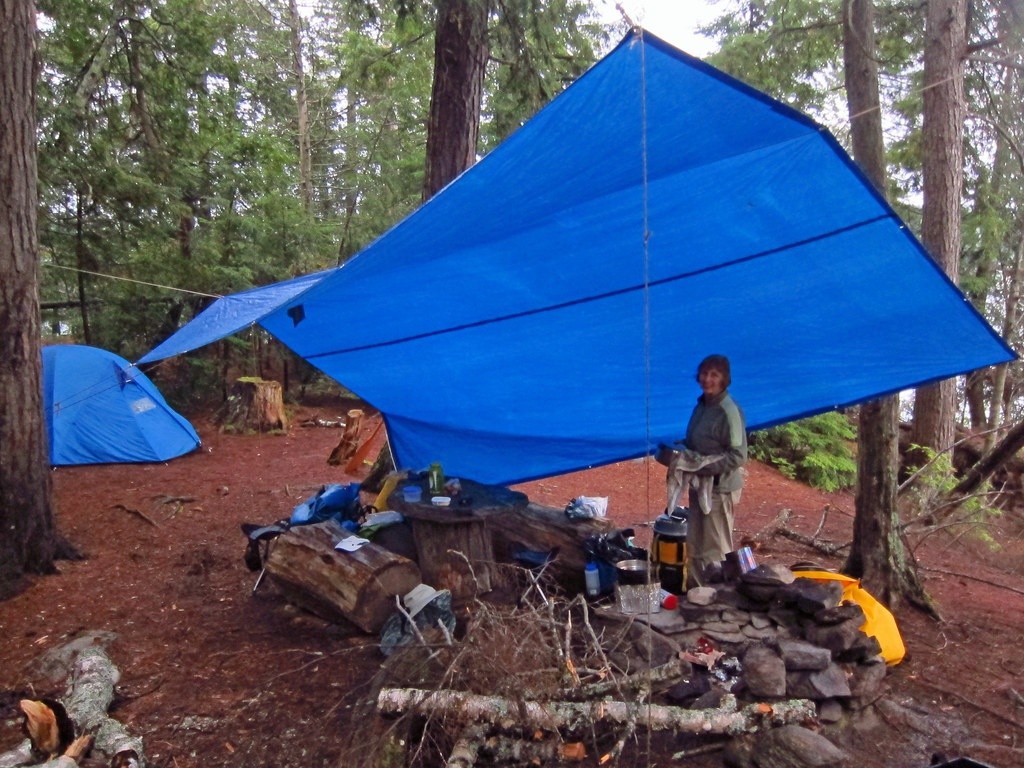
672,354,748,587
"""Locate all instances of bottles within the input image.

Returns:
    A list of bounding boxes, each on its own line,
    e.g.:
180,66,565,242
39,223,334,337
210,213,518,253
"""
660,589,677,609
429,464,444,496
585,563,599,594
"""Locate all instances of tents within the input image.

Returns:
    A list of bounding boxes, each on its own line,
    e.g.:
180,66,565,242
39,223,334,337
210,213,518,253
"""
127,23,1016,489
40,343,202,466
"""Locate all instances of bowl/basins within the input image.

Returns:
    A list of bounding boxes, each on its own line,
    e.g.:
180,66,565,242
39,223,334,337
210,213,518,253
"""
404,486,422,502
617,560,648,584
